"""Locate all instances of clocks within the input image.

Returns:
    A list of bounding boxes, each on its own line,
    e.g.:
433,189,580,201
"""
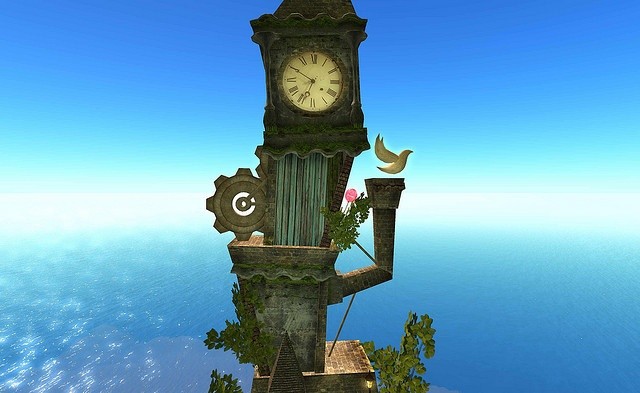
276,45,349,116
